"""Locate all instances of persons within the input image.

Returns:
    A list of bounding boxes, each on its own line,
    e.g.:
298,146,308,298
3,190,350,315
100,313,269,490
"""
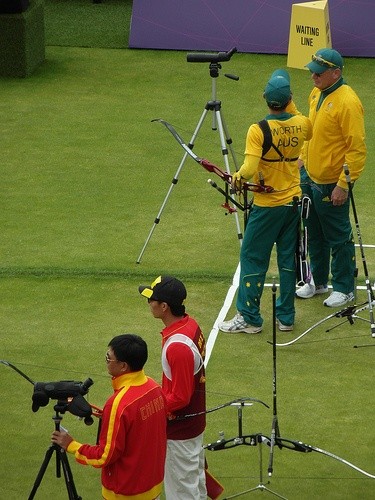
296,49,367,308
50,334,169,500
138,275,209,500
217,69,313,334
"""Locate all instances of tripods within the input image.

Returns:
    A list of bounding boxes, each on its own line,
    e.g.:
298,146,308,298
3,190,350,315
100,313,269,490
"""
26,411,82,500
136,66,244,263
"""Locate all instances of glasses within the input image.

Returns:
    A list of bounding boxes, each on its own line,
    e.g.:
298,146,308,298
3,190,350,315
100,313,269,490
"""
310,69,333,77
105,355,123,364
147,298,157,303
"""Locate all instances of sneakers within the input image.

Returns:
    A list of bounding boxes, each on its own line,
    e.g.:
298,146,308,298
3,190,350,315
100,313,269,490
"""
295,282,328,298
218,314,262,333
323,290,354,307
276,318,295,331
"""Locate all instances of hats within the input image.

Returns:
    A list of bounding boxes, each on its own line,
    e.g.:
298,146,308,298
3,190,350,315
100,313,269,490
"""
304,48,344,74
265,69,291,107
138,275,187,304
